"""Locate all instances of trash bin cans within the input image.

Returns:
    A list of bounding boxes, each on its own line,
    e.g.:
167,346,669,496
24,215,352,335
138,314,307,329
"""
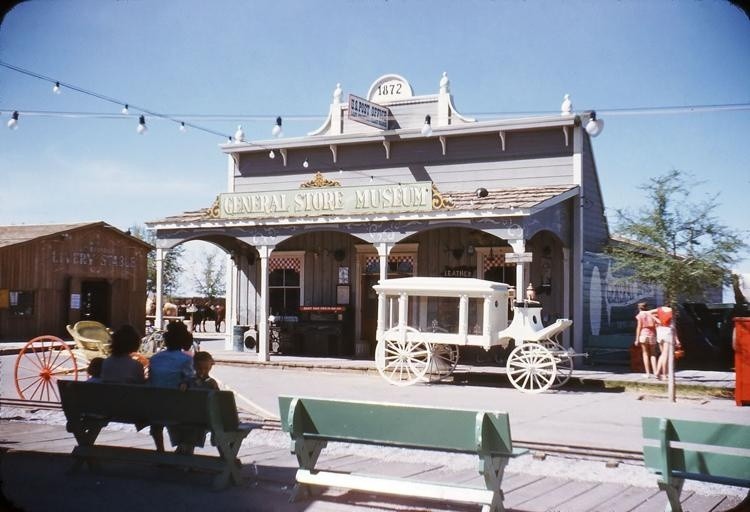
233,326,250,352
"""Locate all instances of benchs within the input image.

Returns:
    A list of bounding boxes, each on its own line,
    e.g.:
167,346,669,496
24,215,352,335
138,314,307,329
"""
56,378,529,512
642,416,750,511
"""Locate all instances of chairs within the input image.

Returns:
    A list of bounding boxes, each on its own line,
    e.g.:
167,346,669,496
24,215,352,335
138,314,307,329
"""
66,320,113,364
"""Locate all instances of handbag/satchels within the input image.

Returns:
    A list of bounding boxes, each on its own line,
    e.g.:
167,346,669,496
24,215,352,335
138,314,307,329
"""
674,345,686,361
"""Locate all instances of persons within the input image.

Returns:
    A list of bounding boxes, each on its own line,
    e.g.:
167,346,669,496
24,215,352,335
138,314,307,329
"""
60,302,225,477
650,301,682,379
634,302,659,379
